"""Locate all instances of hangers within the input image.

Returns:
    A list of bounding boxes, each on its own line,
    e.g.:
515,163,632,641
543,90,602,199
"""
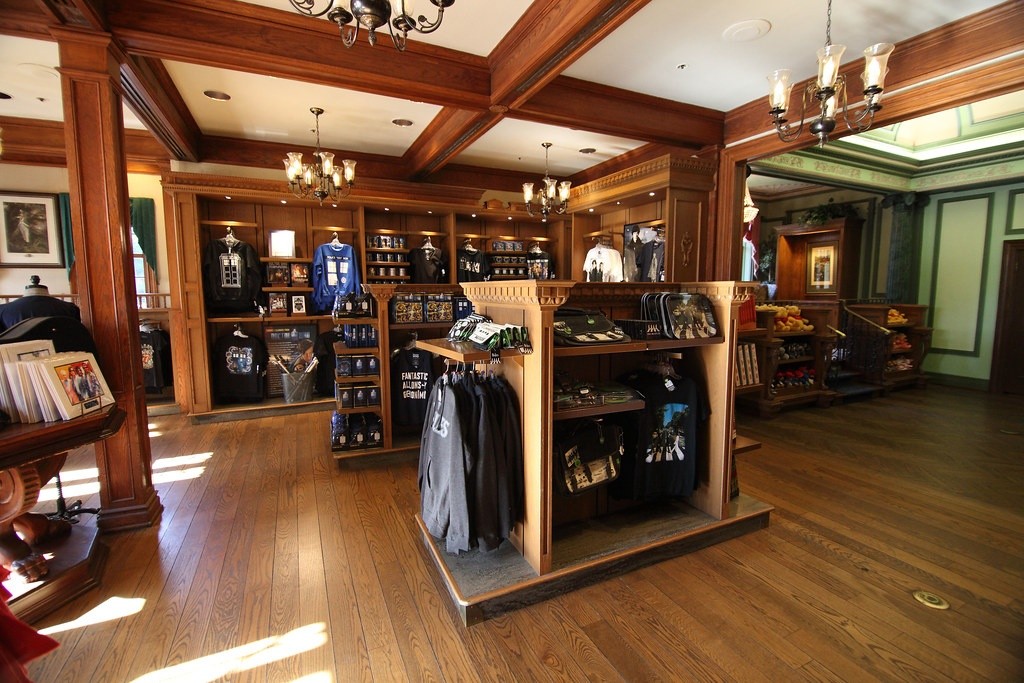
232,322,247,338
401,331,419,351
594,237,613,248
420,237,434,251
527,242,543,253
462,240,478,252
439,358,497,387
329,231,343,247
652,228,665,244
218,227,238,245
139,317,155,333
641,352,682,379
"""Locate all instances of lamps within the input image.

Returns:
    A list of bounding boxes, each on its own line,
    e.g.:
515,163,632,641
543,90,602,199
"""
288,0,455,54
282,105,357,204
767,0,895,149
521,143,572,223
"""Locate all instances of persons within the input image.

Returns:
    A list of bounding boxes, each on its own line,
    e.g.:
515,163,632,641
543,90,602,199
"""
627,225,645,253
0,284,82,336
61,362,101,405
589,260,604,282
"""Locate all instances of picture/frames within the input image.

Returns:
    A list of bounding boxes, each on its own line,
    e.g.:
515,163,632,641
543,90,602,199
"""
0,340,115,423
801,240,840,296
0,190,65,269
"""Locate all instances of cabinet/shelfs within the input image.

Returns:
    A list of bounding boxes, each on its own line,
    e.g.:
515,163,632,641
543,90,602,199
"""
387,285,472,453
406,282,737,574
738,302,935,413
331,282,387,459
189,195,574,410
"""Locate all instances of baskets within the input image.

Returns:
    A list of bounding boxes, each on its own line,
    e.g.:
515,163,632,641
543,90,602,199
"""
282,372,313,403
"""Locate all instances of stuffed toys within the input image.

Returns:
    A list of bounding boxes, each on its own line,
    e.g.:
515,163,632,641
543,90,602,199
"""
887,309,907,323
755,304,815,333
773,366,816,389
890,334,911,350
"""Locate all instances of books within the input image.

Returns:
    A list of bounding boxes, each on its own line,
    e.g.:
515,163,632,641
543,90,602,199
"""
737,294,757,330
735,341,760,387
